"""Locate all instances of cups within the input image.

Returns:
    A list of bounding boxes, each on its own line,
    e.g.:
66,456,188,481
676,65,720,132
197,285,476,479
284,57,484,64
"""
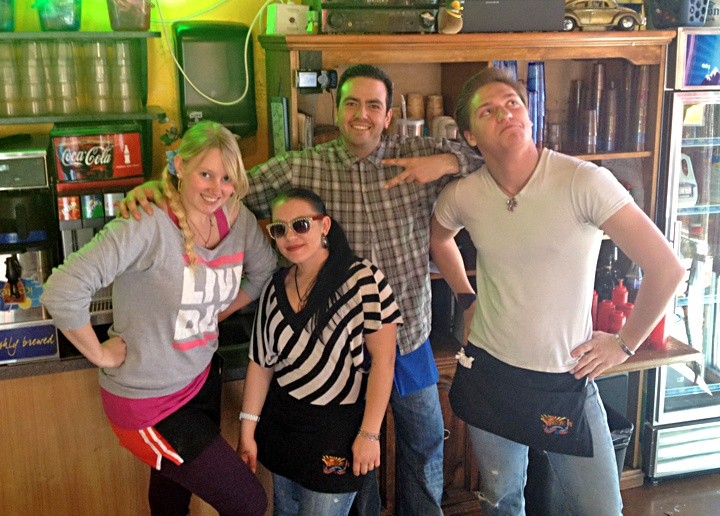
493,59,654,161
0,40,148,118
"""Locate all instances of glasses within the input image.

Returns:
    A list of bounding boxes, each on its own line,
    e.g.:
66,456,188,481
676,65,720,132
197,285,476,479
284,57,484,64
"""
265,214,325,239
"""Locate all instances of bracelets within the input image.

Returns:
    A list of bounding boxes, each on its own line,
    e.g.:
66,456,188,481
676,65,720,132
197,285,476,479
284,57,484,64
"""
615,331,635,356
239,410,261,423
356,427,381,441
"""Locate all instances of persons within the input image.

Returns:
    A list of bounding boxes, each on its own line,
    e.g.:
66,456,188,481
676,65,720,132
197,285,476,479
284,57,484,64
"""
238,184,405,516
427,64,686,514
117,65,486,515
39,120,271,516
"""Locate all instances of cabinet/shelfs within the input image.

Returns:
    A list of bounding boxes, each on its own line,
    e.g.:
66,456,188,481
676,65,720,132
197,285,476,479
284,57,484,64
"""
255,29,677,354
0,31,167,126
378,371,481,515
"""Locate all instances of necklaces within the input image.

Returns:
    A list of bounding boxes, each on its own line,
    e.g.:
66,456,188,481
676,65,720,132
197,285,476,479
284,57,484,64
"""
294,264,309,310
488,146,540,212
186,216,214,249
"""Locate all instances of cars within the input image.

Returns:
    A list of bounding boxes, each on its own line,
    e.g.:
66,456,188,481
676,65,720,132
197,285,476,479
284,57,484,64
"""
563,0,646,31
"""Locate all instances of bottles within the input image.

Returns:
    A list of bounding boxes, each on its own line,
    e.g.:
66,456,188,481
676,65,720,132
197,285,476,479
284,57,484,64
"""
581,245,667,350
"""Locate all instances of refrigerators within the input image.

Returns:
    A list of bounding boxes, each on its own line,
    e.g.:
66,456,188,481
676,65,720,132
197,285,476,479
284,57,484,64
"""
592,30,720,485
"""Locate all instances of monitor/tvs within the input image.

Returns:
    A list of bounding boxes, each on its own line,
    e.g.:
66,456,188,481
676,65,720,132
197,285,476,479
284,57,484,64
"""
171,19,257,139
